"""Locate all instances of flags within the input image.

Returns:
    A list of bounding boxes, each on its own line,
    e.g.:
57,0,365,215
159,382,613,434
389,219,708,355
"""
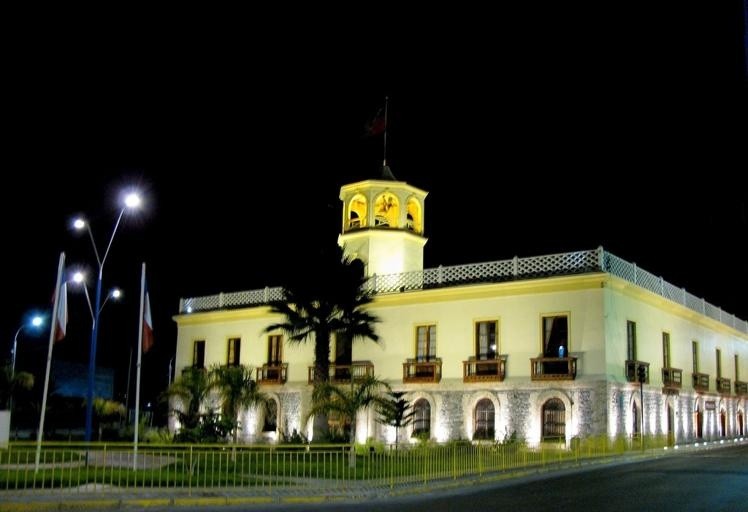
50,260,69,341
142,270,154,353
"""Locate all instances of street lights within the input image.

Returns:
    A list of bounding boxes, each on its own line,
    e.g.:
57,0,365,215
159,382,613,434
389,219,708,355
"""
11,317,43,378
72,193,140,440
